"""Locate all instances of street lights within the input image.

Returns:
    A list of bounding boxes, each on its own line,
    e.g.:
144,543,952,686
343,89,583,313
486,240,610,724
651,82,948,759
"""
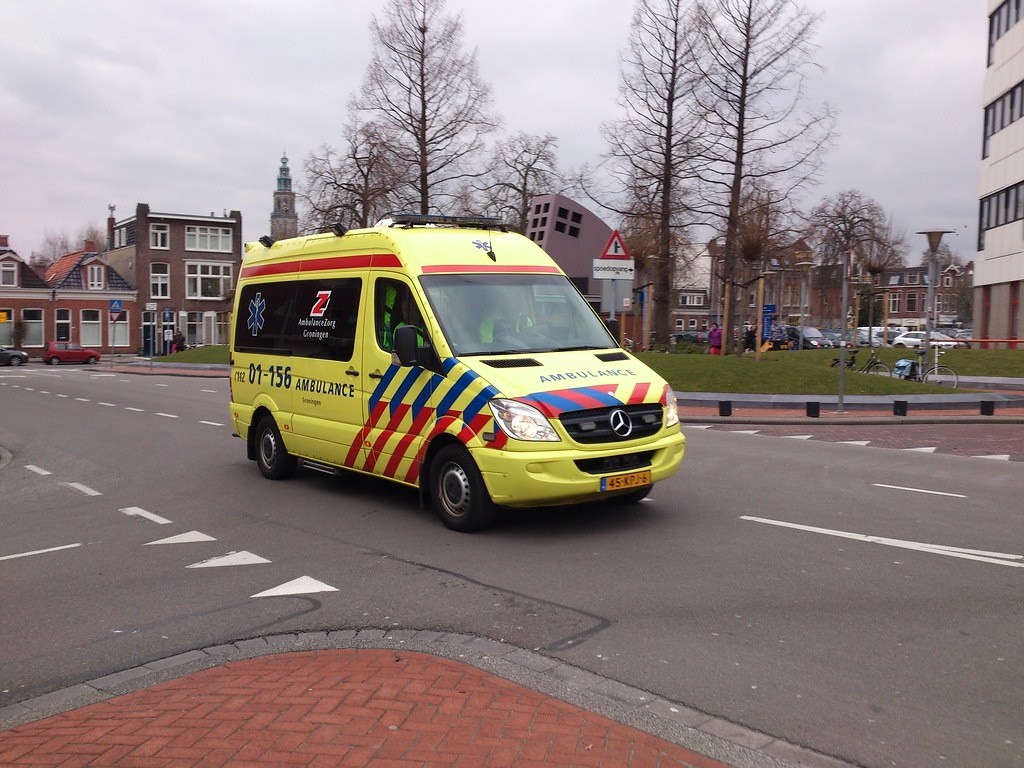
915,230,956,372
763,271,778,305
794,261,817,350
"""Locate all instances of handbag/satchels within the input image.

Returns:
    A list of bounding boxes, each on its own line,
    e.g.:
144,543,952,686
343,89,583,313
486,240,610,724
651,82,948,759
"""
893,360,911,376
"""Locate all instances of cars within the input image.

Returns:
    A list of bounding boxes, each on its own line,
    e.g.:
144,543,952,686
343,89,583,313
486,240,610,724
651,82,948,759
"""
931,328,972,347
820,327,907,348
0,348,28,366
42,341,101,365
892,331,960,349
787,326,834,350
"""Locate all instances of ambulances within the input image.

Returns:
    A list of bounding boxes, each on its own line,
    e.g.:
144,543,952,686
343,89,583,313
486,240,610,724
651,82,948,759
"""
228,214,688,532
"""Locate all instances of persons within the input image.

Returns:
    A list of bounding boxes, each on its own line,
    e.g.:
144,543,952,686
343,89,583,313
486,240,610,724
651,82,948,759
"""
170,332,185,353
708,323,722,355
480,281,533,343
768,318,788,350
743,323,757,352
392,287,478,351
620,332,633,353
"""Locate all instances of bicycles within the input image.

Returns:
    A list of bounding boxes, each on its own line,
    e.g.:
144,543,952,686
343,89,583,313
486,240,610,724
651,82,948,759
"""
831,341,892,377
898,339,959,388
